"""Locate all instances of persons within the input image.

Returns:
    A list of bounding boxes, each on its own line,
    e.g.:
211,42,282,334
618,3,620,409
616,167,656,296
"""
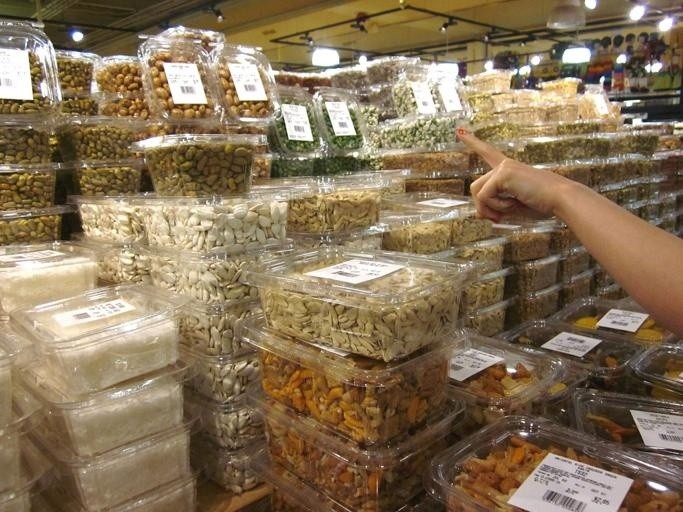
452,127,683,338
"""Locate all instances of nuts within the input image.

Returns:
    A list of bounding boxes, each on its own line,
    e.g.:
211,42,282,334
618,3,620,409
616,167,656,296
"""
1,26,683,512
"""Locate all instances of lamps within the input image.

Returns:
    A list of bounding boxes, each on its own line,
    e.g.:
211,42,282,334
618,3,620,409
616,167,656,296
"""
545,1,586,31
